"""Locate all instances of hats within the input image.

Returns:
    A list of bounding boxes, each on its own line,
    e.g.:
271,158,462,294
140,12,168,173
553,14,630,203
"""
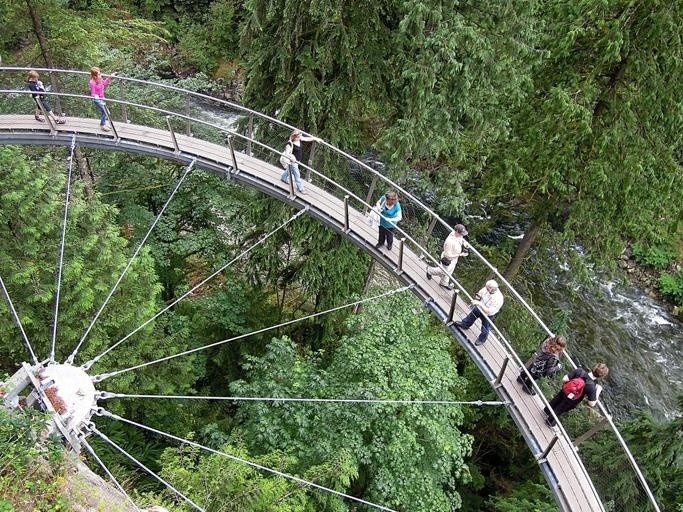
455,224,468,236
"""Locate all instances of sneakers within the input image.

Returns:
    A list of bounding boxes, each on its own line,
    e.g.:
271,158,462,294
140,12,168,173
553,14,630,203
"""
542,409,559,432
475,339,484,345
455,321,462,326
439,283,452,290
281,178,307,195
426,266,432,279
517,377,536,395
35,117,44,122
101,124,113,132
56,118,66,124
376,243,392,250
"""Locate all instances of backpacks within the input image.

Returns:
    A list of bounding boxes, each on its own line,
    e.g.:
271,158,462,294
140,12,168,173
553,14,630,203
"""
562,369,595,401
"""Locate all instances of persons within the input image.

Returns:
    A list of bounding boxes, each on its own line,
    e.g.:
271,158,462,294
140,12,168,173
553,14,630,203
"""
517,336,567,395
89,67,114,132
543,362,610,428
426,223,473,297
26,71,67,124
372,191,402,250
280,128,323,197
454,279,506,346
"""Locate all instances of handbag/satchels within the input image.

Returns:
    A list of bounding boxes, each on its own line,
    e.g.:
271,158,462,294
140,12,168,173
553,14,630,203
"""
441,257,451,266
280,140,293,169
367,206,380,227
